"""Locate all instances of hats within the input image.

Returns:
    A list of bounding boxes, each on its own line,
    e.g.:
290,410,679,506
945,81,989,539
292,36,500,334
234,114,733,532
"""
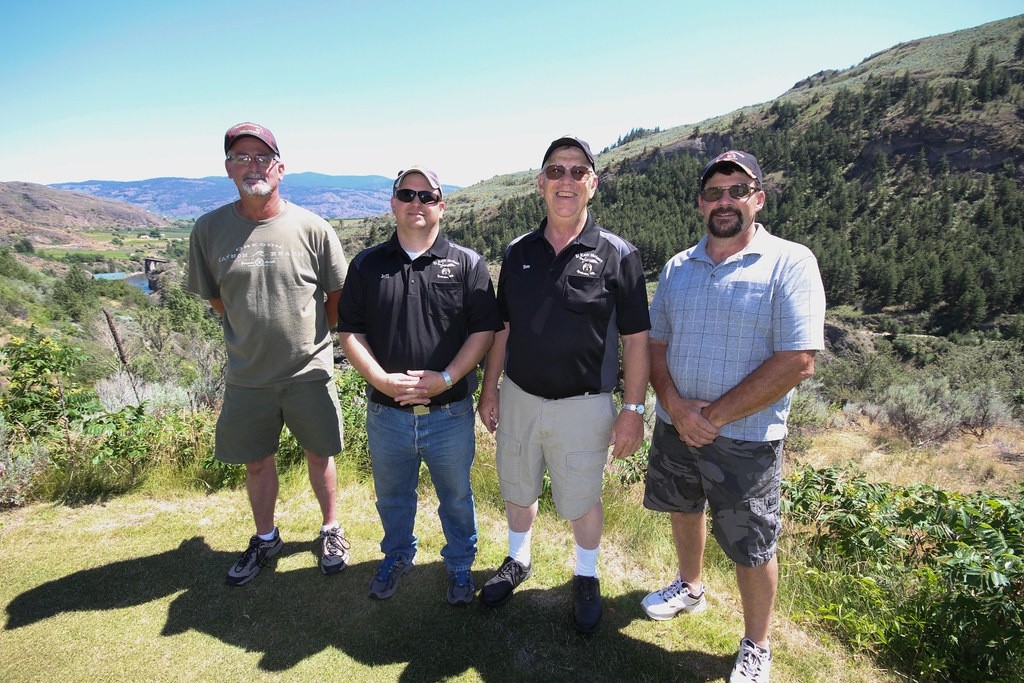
700,150,762,190
393,167,442,198
225,122,280,158
541,135,595,175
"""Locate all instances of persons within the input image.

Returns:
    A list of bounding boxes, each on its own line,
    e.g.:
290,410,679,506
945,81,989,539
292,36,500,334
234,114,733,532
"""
188,122,349,585
640,149,826,682
477,135,652,634
338,168,506,608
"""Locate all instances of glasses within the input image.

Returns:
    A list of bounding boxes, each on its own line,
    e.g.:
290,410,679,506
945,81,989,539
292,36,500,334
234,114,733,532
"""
395,188,441,205
700,184,758,202
541,164,591,181
226,154,275,168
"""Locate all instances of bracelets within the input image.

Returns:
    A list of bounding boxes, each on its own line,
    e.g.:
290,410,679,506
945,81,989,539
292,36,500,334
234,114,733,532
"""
439,369,453,390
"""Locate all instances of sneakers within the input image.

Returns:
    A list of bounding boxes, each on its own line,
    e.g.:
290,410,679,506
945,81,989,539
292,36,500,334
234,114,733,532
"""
227,524,283,586
729,636,772,683
640,576,705,620
447,568,474,605
573,574,601,632
481,556,532,606
368,555,414,600
319,523,350,575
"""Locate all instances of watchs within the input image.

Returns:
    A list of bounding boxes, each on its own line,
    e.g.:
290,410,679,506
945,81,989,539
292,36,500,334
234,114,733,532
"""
622,403,645,415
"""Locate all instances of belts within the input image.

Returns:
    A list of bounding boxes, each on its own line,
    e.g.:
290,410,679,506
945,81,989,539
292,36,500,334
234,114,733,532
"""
400,403,441,415
